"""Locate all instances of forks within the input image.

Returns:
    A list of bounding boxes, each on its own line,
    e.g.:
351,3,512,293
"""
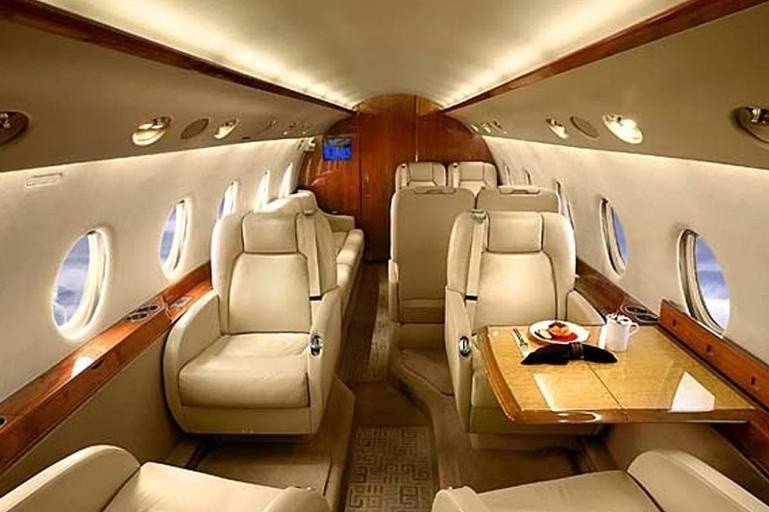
513,328,528,356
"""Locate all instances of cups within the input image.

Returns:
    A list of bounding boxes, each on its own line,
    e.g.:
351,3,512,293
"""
605,315,639,351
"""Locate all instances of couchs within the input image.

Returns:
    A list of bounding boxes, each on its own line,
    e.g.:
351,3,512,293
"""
389,158,758,438
431,447,767,512
267,190,364,314
163,210,342,449
1,444,331,510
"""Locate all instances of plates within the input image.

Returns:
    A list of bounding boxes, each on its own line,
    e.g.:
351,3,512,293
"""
530,320,589,345
